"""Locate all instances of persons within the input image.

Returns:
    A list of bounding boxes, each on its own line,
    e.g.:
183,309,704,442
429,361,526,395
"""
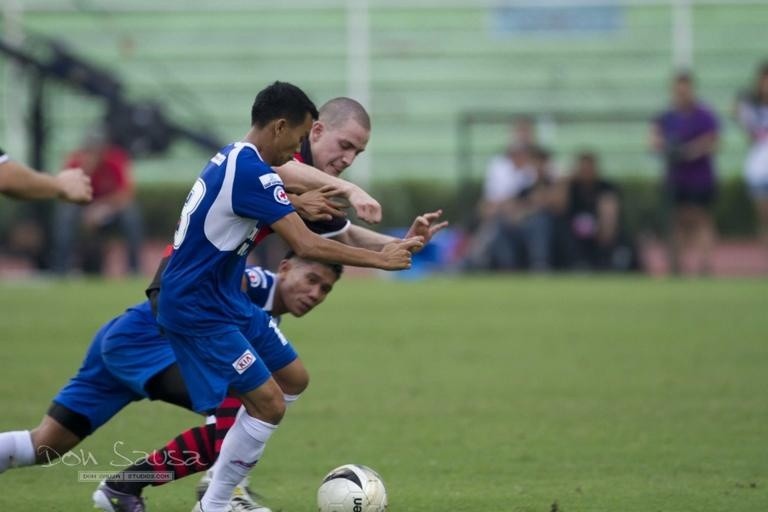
2,151,91,207
158,82,427,512
51,118,145,275
94,93,449,511
1,246,344,499
650,71,722,272
470,112,647,273
730,63,768,245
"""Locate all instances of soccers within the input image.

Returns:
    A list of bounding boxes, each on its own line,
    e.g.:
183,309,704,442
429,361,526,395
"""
318,463,387,512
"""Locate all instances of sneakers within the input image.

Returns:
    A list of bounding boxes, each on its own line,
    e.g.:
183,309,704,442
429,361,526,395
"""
190,474,272,512
92,477,146,512
